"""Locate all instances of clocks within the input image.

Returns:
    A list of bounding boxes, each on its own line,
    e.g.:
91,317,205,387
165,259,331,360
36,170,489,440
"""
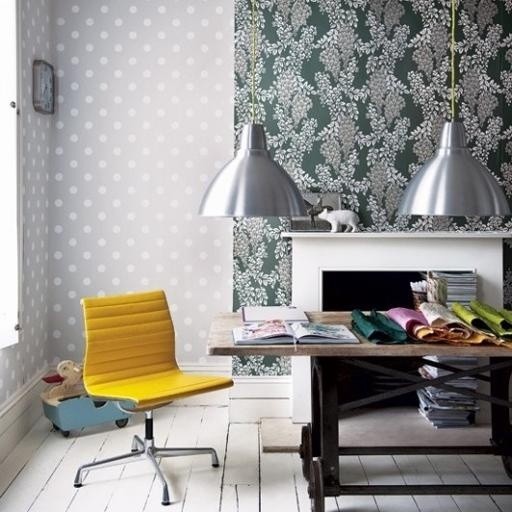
32,59,58,115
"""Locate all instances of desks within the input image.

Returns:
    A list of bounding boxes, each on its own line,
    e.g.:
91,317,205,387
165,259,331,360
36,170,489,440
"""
206,306,512,512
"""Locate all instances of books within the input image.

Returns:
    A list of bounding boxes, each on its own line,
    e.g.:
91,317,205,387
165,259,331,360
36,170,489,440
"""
232,320,361,344
241,303,310,325
414,268,479,431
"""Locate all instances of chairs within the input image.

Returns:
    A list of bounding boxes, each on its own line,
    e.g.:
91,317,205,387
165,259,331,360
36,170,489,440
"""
72,289,234,505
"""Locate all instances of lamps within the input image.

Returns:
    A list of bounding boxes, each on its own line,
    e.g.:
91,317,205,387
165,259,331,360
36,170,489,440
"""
196,0,313,220
395,1,512,218
